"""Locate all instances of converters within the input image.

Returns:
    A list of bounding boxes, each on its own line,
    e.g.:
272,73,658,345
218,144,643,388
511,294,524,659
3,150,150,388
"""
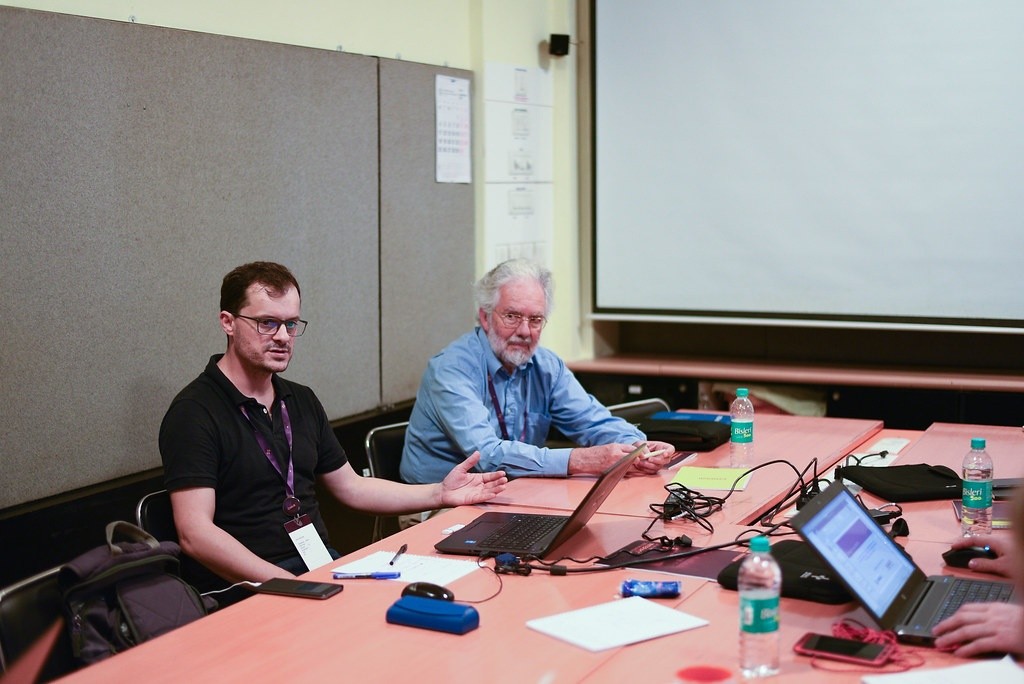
663,491,690,515
867,508,890,525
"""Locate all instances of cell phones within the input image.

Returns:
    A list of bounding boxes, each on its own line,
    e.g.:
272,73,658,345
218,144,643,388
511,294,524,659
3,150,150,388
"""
253,577,344,599
794,633,896,666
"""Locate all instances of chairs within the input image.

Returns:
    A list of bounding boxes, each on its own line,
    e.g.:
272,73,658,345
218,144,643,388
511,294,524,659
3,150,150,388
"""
363,422,407,547
601,397,671,425
136,490,180,550
0,563,71,678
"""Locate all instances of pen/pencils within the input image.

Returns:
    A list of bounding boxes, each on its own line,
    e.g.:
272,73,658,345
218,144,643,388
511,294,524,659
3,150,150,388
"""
641,449,666,459
333,572,401,579
390,544,408,565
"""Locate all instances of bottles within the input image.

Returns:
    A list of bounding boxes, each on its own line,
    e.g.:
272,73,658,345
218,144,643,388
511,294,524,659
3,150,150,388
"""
736,537,780,677
728,388,753,469
962,438,995,552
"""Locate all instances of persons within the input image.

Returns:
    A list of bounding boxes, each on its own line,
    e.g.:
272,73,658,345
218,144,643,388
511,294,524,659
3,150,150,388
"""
932,534,1024,657
158,261,507,591
397,258,676,530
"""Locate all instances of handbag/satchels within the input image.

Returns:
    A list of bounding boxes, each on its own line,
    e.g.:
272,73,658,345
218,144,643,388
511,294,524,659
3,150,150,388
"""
63,520,219,668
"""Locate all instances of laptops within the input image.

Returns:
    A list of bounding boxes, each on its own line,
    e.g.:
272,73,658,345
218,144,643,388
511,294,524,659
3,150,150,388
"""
434,443,647,560
789,479,1022,659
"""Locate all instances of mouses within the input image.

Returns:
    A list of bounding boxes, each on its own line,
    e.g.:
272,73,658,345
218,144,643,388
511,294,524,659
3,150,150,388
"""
942,545,1000,568
401,582,455,603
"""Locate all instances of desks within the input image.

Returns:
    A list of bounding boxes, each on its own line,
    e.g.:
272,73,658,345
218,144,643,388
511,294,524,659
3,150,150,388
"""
49,410,1024,683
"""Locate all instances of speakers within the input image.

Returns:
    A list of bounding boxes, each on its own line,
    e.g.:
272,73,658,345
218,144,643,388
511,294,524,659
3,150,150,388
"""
549,34,570,56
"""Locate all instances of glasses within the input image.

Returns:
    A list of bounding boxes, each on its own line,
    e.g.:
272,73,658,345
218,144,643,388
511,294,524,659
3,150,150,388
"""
232,314,308,337
493,304,548,330
